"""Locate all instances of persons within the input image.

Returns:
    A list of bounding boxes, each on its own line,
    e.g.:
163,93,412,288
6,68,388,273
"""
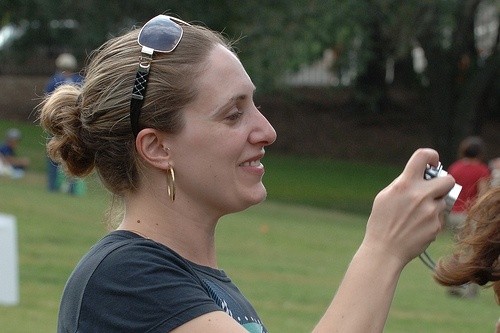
447,137,490,225
41,53,87,194
38,16,454,333
0,129,31,178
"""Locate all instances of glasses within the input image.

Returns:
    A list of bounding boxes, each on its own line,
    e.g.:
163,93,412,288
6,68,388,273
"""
130,15,191,151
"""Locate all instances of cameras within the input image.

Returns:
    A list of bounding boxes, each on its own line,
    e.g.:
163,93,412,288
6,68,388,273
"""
423,161,463,211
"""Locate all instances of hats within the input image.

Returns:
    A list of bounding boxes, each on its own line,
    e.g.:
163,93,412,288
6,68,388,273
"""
56,54,77,72
8,128,21,139
